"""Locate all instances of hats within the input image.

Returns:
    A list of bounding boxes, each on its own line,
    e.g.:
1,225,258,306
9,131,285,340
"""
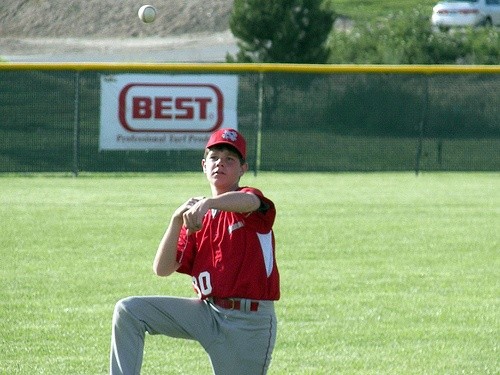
206,128,247,159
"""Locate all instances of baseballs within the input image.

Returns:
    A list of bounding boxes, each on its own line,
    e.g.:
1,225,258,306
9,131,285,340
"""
138,5,158,23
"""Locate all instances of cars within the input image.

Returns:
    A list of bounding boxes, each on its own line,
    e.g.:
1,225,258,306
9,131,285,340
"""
430,0,500,33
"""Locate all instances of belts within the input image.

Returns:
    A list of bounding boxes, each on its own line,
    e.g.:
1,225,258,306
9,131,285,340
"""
214,297,257,311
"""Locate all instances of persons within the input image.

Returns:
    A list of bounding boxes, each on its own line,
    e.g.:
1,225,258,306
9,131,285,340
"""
110,128,280,375
